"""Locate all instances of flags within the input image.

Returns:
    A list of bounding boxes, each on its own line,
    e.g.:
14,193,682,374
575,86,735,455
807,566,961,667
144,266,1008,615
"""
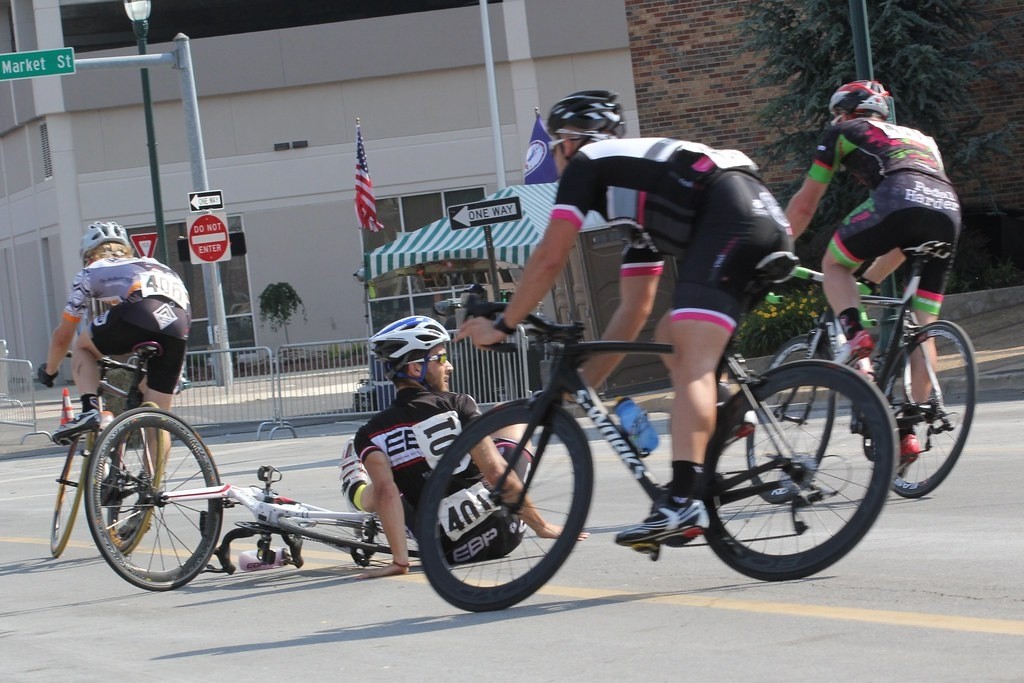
525,117,560,185
354,126,384,232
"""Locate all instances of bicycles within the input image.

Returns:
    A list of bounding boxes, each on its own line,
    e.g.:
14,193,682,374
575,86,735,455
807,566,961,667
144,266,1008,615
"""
201,464,419,574
36,337,225,591
744,231,979,507
413,248,903,615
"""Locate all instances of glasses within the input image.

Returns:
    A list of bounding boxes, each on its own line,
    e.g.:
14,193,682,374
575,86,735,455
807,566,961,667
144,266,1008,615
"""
546,137,581,157
404,350,449,364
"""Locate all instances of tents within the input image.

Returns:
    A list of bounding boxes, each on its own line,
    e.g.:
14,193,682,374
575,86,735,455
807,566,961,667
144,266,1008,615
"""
363,183,612,340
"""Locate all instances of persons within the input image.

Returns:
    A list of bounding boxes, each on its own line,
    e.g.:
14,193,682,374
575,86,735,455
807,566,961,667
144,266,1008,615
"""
451,89,795,545
455,283,485,329
338,315,590,579
38,221,192,541
784,79,960,479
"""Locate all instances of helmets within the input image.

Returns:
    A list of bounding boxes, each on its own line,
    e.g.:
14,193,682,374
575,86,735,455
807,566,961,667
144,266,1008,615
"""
368,316,451,364
550,90,626,140
78,222,130,267
829,80,890,119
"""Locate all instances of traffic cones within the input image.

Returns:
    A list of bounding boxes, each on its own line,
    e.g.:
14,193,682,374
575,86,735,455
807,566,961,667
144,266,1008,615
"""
60,386,75,427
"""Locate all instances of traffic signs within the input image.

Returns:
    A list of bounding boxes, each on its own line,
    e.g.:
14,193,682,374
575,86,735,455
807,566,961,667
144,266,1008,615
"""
447,195,523,230
188,189,225,214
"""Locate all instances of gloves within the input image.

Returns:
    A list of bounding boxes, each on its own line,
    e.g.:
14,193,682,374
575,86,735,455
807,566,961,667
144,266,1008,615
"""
37,362,59,388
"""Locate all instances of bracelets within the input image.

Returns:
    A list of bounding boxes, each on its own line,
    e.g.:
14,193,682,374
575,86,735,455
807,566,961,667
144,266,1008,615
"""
393,560,409,568
492,313,516,335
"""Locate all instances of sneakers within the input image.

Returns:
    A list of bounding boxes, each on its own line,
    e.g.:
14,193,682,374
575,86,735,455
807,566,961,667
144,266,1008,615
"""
340,439,367,511
52,409,101,446
715,399,757,449
617,487,709,546
117,509,152,542
832,332,874,368
897,435,920,479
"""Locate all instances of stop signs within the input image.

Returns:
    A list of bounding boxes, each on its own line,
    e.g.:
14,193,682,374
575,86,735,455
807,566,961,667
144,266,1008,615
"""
186,211,232,265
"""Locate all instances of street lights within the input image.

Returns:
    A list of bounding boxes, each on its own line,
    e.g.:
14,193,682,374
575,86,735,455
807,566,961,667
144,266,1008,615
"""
118,0,170,267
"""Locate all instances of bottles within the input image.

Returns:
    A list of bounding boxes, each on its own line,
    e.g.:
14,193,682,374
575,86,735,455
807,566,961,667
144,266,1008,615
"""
99,406,115,452
238,548,291,571
853,357,874,380
613,394,659,451
